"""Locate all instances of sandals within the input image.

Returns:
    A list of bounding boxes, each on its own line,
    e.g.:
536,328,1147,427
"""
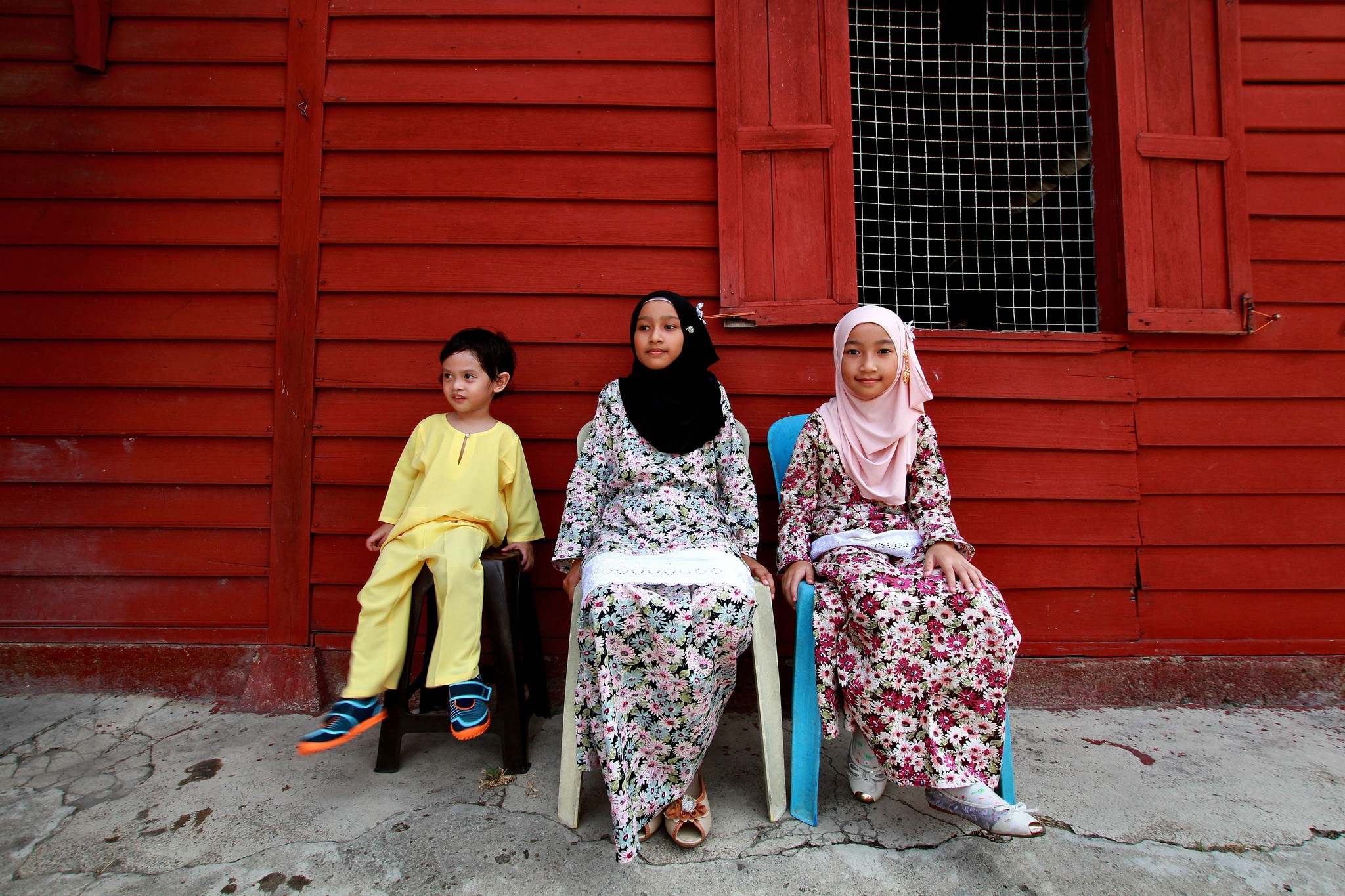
638,811,662,843
672,770,712,849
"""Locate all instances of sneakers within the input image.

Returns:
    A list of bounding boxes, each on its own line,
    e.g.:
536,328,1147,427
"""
449,675,492,741
295,695,388,755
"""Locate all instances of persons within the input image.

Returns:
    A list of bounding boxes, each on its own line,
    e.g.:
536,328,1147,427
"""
295,328,546,757
551,290,773,863
776,305,1044,838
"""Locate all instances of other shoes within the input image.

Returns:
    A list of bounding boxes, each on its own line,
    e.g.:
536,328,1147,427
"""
846,732,887,804
924,786,1045,838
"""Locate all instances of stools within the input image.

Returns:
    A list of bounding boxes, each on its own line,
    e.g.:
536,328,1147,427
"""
372,547,553,775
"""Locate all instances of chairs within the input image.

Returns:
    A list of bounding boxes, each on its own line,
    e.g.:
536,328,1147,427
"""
767,412,1014,828
556,419,786,829
